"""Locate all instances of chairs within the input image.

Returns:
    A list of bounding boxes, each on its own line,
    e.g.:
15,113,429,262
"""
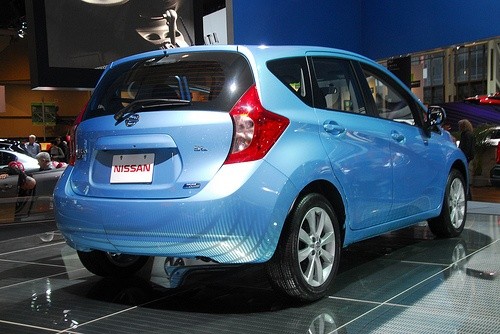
131,70,192,103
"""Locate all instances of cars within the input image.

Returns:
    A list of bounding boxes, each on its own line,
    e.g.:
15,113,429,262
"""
52,43,470,304
474,123,500,177
0,142,69,226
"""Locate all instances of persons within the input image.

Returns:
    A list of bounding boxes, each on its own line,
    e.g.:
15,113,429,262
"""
0,134,69,214
457,120,479,200
